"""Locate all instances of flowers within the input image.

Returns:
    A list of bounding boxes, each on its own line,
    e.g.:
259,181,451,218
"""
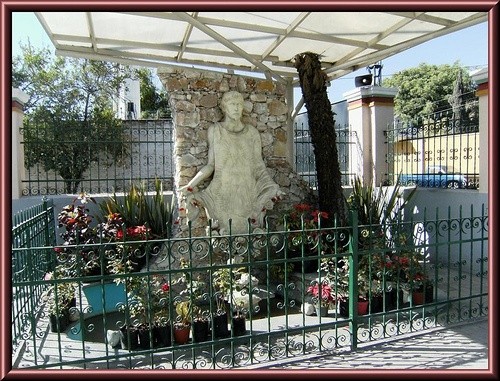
152,275,177,327
285,205,332,240
53,193,154,275
307,252,427,305
226,257,261,316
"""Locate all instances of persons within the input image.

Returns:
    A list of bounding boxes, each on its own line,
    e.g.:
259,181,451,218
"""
176,91,286,232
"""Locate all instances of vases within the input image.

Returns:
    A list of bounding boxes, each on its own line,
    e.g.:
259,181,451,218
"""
314,305,328,317
339,291,403,316
151,325,172,347
232,316,246,337
413,283,434,305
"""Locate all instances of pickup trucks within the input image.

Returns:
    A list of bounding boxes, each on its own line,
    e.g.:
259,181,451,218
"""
400,166,469,190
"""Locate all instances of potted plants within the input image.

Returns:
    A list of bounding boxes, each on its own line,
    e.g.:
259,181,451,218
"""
177,256,208,342
210,267,230,337
174,301,190,345
107,258,155,350
43,275,76,332
